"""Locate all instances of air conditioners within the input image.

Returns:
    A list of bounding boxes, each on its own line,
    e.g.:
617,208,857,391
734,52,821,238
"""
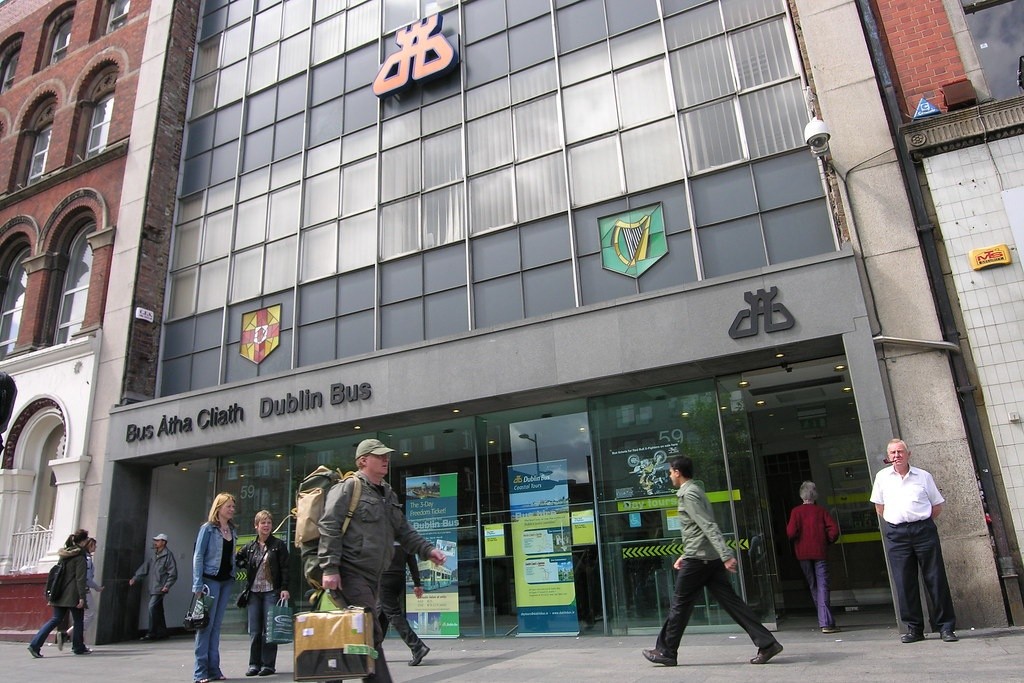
796,406,829,421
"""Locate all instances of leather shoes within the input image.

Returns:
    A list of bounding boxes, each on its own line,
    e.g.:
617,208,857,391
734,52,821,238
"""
940,630,957,641
750,641,783,664
901,633,924,642
643,649,677,665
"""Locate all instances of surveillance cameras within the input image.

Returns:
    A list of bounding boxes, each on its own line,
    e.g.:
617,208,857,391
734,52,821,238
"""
803,120,831,146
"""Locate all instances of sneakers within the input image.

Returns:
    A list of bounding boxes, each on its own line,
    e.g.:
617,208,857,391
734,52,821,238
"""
408,645,429,666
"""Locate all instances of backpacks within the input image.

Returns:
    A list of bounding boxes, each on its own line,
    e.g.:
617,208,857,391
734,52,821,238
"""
46,556,76,601
293,465,362,585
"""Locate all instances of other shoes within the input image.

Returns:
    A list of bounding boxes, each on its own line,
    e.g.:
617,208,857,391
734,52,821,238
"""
258,669,273,675
215,675,226,680
199,678,211,683
77,648,91,654
140,635,153,640
28,646,43,658
57,631,67,651
246,669,258,676
822,626,841,633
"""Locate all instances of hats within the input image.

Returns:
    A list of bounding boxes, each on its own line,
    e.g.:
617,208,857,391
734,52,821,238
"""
355,438,396,456
153,534,168,541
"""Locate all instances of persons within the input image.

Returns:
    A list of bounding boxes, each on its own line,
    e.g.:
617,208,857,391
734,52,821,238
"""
192,492,236,682
869,439,960,642
129,532,177,641
786,481,840,635
236,510,291,676
318,439,446,683
642,456,784,665
28,529,101,658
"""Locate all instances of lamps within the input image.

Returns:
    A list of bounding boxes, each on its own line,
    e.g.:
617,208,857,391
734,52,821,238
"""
940,77,978,110
781,362,793,373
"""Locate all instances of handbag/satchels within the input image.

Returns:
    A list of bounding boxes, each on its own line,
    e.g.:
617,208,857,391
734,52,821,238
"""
266,597,294,643
182,592,209,629
292,589,377,681
236,588,250,607
193,584,215,615
